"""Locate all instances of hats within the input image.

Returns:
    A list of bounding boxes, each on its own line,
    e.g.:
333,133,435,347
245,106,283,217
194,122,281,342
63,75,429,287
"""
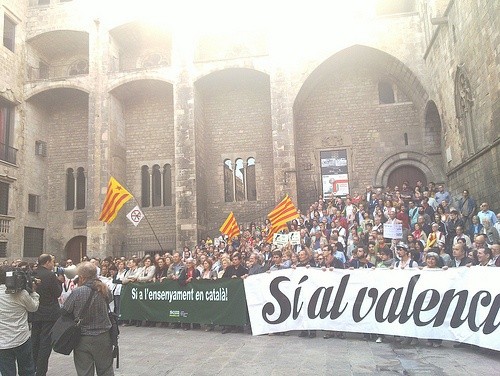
397,242,408,248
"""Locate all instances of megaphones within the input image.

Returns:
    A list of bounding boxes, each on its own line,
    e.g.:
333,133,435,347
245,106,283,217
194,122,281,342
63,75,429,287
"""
57,265,77,279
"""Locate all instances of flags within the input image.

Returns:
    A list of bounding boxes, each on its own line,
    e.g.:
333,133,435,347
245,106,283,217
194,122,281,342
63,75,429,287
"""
265,191,301,243
218,210,240,245
97,176,133,224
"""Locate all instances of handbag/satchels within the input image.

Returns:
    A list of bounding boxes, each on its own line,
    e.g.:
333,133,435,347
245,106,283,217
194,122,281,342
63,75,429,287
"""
108,312,120,346
48,313,80,355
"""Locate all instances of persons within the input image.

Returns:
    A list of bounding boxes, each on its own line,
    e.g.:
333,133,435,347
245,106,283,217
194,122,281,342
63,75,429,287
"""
29,254,65,376
63,261,116,376
0,178,500,351
0,265,40,376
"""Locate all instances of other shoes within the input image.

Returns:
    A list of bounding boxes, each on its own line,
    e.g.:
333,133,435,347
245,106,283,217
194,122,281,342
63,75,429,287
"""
126,319,480,351
